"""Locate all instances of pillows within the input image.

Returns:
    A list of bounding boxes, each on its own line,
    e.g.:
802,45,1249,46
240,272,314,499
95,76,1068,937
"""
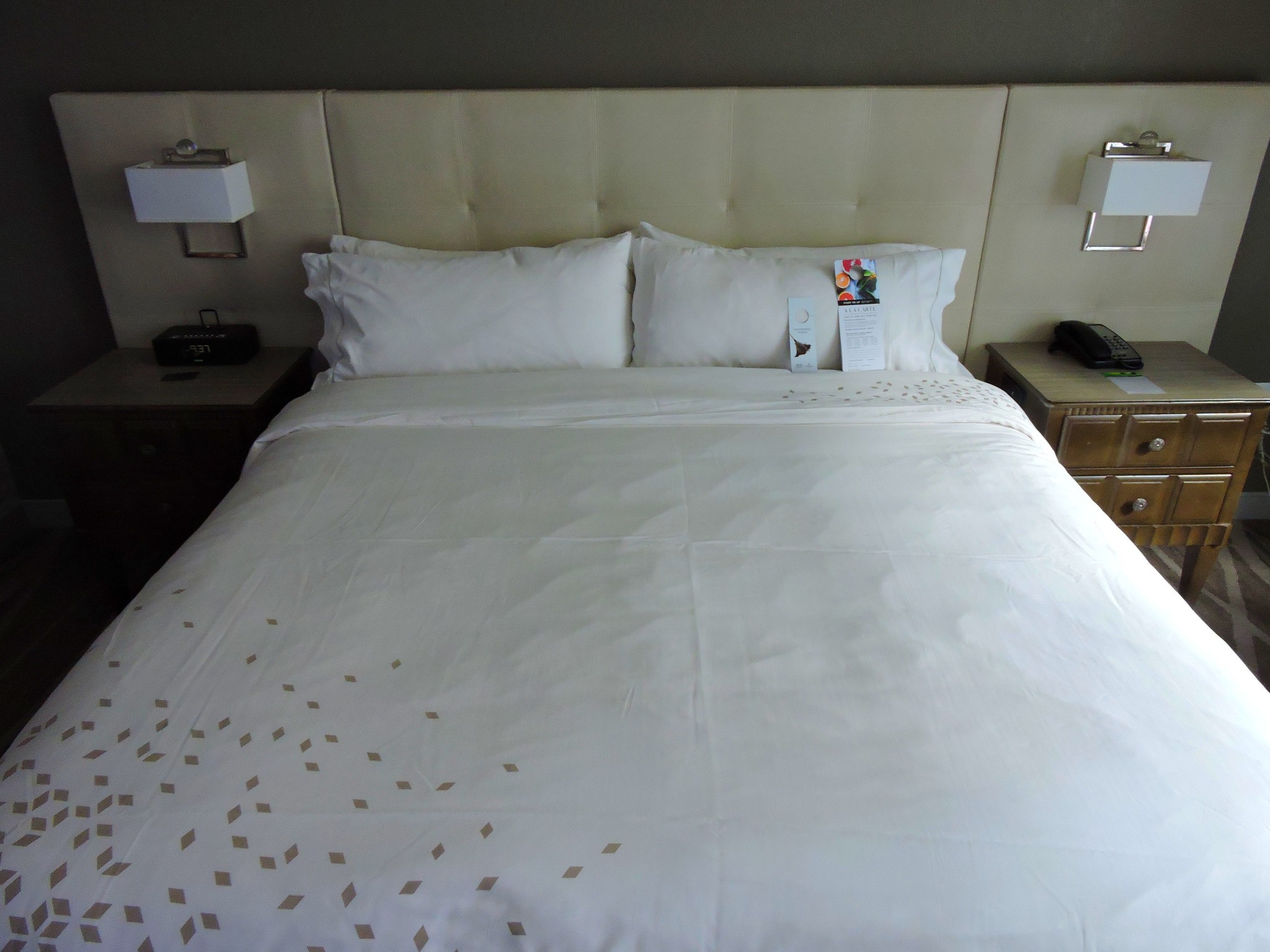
630,238,967,371
295,236,631,384
329,233,480,256
635,220,932,255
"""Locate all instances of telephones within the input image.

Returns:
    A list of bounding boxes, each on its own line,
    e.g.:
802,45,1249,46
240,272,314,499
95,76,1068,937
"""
1054,320,1144,370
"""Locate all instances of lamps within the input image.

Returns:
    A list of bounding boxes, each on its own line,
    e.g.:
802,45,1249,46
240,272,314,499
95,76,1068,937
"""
1075,131,1211,252
120,141,249,259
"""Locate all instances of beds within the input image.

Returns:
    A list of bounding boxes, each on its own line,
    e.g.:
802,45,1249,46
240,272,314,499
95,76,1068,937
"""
1,347,1270,949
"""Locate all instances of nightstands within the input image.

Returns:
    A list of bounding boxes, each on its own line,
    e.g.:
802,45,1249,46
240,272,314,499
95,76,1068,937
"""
985,341,1268,603
27,348,275,582
